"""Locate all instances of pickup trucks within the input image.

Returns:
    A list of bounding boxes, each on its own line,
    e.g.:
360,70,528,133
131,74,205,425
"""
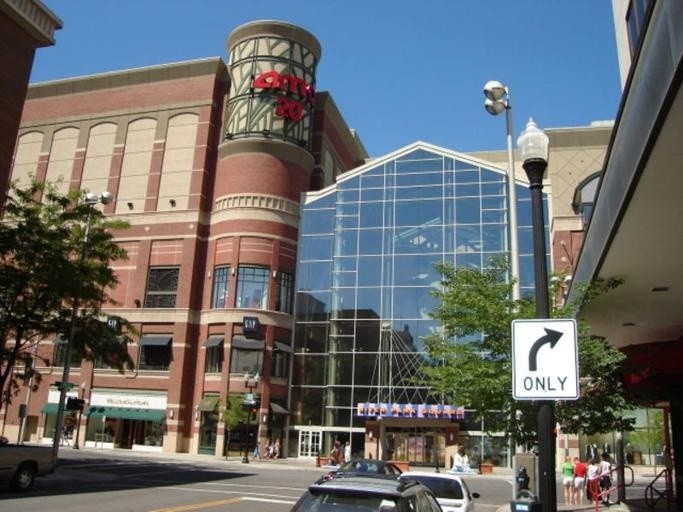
0,442,57,492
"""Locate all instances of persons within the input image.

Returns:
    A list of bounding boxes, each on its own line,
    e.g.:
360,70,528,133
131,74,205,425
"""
252,437,281,461
343,440,350,462
561,452,612,506
61,425,70,447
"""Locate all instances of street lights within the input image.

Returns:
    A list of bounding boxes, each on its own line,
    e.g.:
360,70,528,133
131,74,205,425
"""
73,380,87,449
53,191,113,465
242,371,261,463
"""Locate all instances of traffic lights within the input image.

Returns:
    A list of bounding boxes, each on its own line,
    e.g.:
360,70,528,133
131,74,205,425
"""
66,396,85,412
252,407,257,420
18,403,27,418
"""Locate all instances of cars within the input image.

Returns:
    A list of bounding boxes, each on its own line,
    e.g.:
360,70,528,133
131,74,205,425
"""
289,458,481,512
